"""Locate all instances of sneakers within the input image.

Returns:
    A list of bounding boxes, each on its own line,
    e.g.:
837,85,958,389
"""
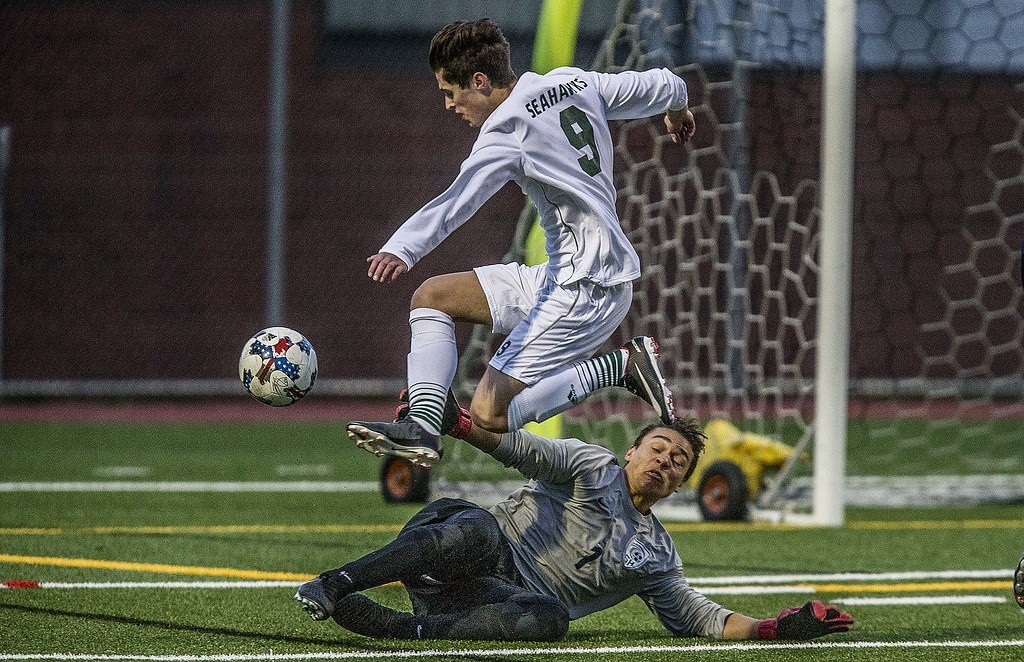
619,336,678,425
330,593,428,641
346,415,445,470
293,569,358,621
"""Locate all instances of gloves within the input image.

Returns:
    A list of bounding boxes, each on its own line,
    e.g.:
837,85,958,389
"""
393,386,472,440
758,601,854,640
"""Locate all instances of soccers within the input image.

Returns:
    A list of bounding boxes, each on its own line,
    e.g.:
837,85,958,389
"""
237,325,320,407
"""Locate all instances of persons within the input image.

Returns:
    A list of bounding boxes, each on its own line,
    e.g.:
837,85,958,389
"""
347,19,695,471
293,386,857,643
